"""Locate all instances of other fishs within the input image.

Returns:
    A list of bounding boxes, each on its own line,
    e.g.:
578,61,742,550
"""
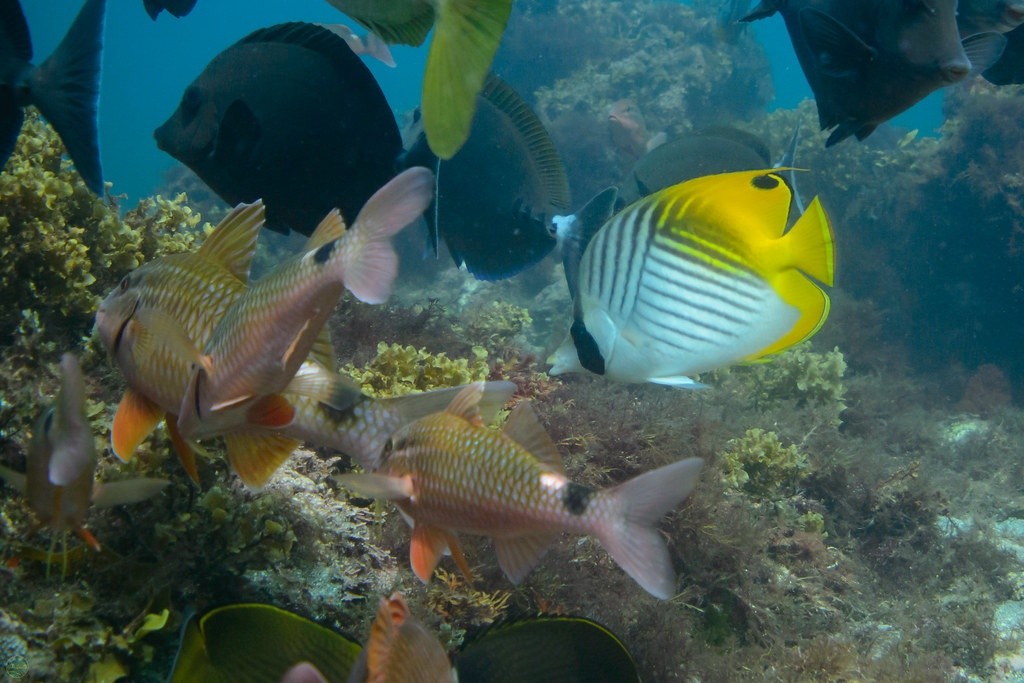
0,0,1024,682
